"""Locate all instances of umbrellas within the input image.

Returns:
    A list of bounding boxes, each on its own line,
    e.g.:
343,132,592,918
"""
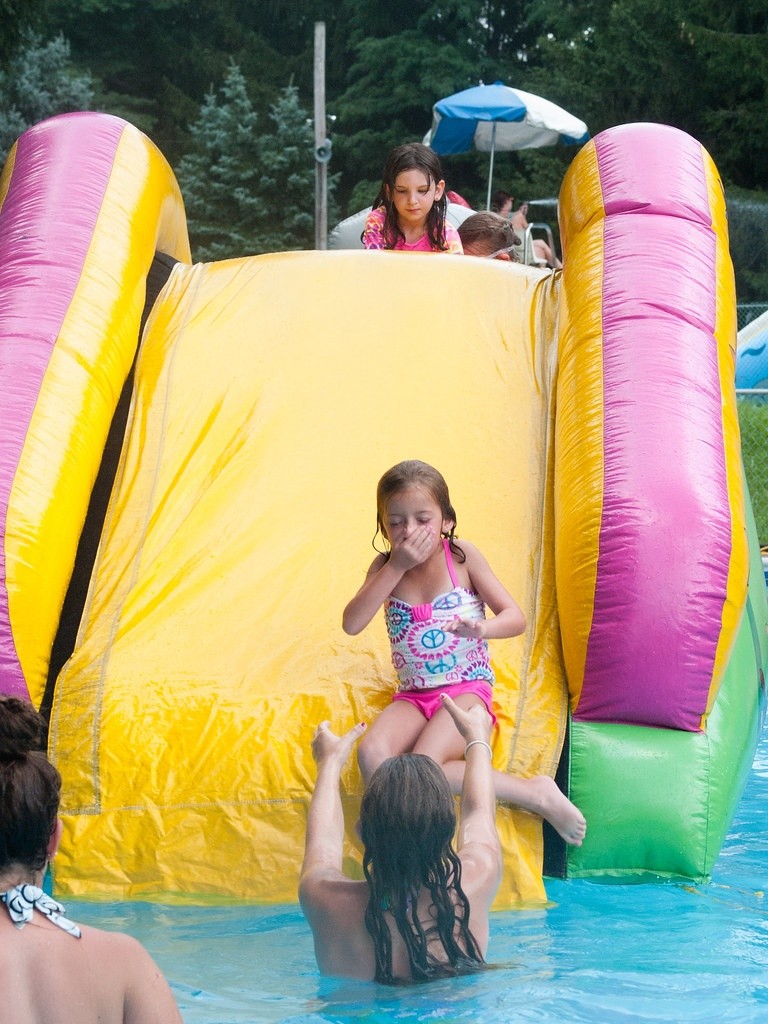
420,80,592,213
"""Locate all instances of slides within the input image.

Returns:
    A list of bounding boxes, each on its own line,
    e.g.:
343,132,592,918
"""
0,109,768,914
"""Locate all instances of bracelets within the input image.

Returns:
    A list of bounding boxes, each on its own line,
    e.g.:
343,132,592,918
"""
465,740,492,763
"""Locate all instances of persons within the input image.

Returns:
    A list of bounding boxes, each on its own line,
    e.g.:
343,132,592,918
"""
343,460,589,851
297,692,502,981
360,143,563,270
0,696,184,1024
